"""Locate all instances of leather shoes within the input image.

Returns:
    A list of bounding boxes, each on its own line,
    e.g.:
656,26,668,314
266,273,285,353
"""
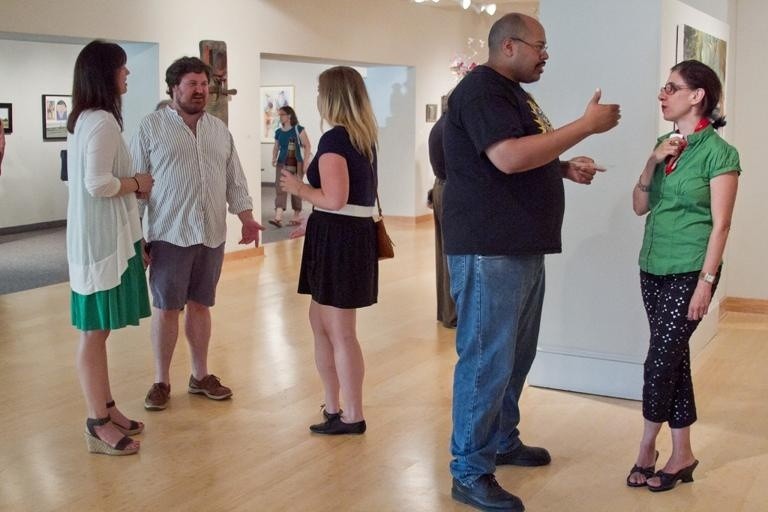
451,472,526,512
494,441,551,466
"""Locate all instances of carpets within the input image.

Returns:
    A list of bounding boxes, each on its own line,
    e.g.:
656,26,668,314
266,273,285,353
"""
0,184,317,297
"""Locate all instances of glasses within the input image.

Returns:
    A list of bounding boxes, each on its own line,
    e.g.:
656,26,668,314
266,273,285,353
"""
509,35,549,56
659,82,697,96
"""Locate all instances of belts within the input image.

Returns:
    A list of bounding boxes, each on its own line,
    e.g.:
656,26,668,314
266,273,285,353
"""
434,176,448,186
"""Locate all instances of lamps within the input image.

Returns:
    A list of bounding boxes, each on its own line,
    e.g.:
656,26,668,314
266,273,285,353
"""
458,0,502,16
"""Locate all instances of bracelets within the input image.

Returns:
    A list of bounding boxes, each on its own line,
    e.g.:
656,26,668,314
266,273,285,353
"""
132,176,141,194
638,173,651,194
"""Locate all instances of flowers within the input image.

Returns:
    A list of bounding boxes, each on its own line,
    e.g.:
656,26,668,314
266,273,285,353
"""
448,36,487,84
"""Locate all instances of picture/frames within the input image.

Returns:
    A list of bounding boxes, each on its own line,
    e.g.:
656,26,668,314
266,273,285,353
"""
259,84,296,146
40,93,74,143
0,101,13,136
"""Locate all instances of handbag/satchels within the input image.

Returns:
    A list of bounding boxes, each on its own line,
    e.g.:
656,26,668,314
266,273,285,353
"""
299,151,315,173
375,218,396,261
60,149,68,181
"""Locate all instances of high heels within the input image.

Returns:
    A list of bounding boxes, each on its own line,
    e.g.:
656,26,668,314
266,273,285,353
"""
84,412,141,456
105,400,145,437
647,458,699,492
625,449,659,487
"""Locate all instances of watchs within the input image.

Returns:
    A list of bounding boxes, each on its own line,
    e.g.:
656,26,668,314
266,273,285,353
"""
695,270,717,284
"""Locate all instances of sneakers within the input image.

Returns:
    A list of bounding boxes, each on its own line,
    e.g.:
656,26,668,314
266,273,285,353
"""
320,403,344,419
187,374,233,401
143,381,170,411
309,415,368,435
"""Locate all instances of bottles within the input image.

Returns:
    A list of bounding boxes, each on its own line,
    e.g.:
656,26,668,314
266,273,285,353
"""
282,139,297,175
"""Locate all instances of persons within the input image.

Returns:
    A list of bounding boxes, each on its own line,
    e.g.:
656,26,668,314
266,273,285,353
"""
65,39,155,455
267,105,312,228
427,87,458,330
279,64,379,435
439,10,622,512
126,54,265,411
56,99,68,121
47,101,56,120
0,117,6,177
623,59,743,492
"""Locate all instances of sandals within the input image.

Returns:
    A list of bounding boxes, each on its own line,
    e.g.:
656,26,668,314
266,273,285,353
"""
267,217,285,228
285,219,304,226
